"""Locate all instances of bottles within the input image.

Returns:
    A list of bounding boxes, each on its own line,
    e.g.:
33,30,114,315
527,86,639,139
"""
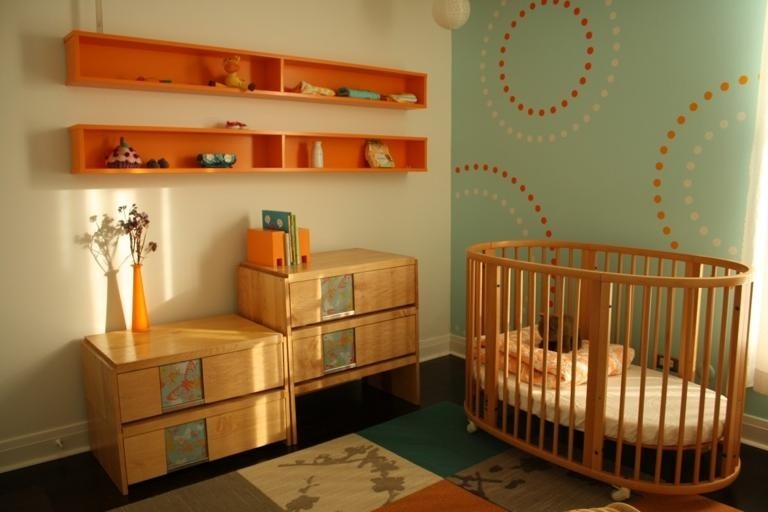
311,141,324,168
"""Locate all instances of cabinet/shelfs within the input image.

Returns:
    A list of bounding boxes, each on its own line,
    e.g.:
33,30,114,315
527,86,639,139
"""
81,314,292,498
68,124,426,174
238,248,421,445
63,31,427,109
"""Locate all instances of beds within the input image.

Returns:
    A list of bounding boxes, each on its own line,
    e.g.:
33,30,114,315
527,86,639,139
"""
464,239,756,501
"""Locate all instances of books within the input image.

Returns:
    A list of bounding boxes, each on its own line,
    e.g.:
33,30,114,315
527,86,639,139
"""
261,208,301,267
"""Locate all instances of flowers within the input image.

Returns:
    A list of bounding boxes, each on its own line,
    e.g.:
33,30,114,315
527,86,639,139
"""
116,202,158,263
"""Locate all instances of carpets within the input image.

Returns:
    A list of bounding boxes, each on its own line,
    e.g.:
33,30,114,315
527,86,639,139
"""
103,402,748,511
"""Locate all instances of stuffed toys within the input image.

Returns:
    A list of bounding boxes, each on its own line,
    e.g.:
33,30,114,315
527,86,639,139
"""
209,53,258,92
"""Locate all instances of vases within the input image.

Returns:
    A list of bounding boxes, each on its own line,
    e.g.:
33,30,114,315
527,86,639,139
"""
130,265,149,332
